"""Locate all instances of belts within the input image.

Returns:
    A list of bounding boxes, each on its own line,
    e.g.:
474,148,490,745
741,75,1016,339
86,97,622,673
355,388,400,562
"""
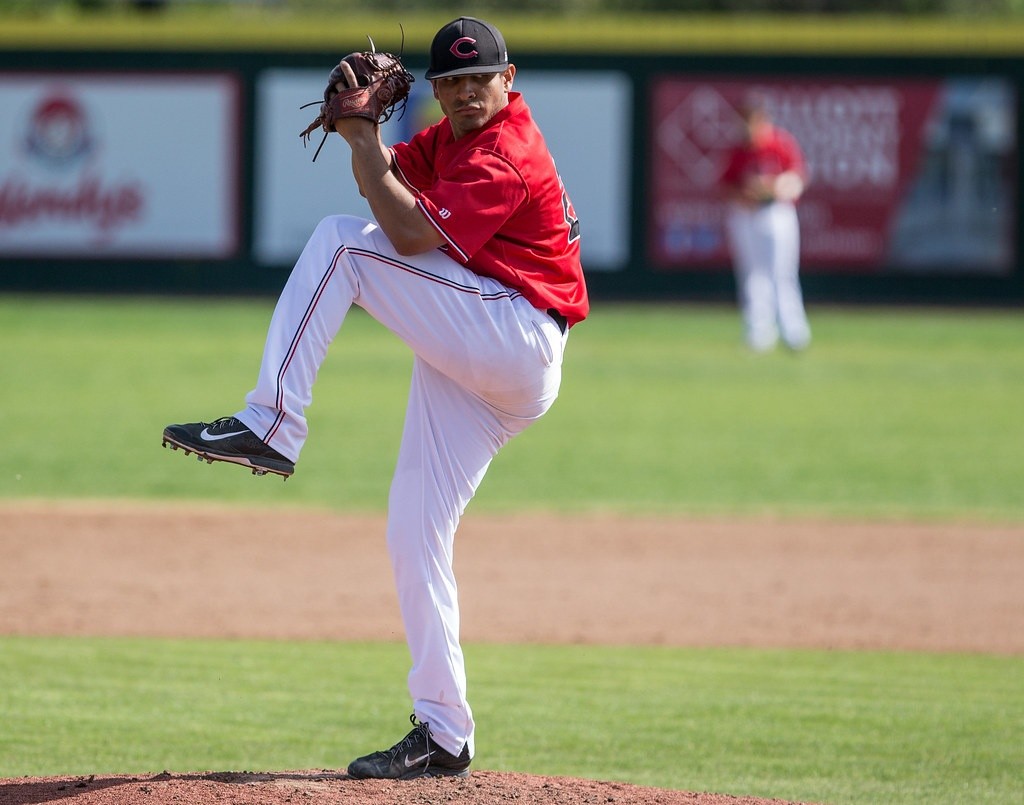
547,309,569,336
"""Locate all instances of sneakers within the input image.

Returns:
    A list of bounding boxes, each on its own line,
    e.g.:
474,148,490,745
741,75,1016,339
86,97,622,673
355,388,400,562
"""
161,416,295,482
347,714,472,780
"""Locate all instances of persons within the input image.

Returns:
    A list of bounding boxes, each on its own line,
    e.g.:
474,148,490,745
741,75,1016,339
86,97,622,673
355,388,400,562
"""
717,91,811,352
162,18,589,781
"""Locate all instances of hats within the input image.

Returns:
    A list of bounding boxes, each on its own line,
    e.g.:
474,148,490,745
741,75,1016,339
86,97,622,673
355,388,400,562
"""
424,16,508,80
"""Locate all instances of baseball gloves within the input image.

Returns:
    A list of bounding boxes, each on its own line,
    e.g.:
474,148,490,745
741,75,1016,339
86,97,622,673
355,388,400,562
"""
321,52,415,133
